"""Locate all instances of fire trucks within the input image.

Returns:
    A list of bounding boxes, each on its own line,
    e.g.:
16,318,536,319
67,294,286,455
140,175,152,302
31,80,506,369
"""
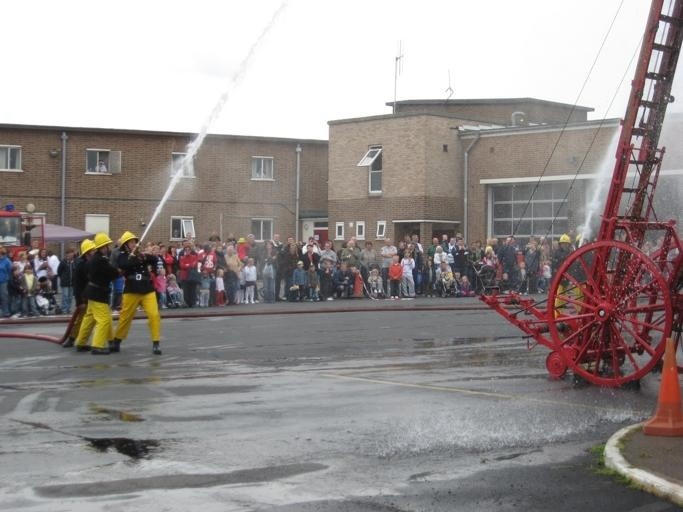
0,204,46,261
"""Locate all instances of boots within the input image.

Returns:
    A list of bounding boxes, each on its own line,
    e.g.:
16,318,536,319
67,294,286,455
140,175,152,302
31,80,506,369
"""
62,336,121,354
152,341,161,354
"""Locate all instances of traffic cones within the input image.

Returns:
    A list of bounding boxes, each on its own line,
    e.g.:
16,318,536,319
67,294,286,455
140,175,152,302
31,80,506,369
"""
642,337,683,436
350,270,363,299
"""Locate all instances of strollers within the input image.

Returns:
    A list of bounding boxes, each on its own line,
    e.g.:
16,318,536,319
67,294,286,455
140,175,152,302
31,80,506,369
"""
472,264,500,295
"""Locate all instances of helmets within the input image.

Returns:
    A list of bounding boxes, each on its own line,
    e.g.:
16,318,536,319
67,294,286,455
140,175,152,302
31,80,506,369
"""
558,233,571,245
80,233,113,256
118,231,139,251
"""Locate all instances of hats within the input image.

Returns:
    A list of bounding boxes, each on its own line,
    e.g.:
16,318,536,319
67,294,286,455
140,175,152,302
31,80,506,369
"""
237,237,246,243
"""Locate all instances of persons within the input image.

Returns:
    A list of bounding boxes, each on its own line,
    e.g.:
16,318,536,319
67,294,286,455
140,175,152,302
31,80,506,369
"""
256,230,676,303
0,234,263,319
62,231,162,355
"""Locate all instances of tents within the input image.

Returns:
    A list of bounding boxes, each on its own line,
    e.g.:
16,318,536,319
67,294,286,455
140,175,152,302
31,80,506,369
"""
22,223,96,242
